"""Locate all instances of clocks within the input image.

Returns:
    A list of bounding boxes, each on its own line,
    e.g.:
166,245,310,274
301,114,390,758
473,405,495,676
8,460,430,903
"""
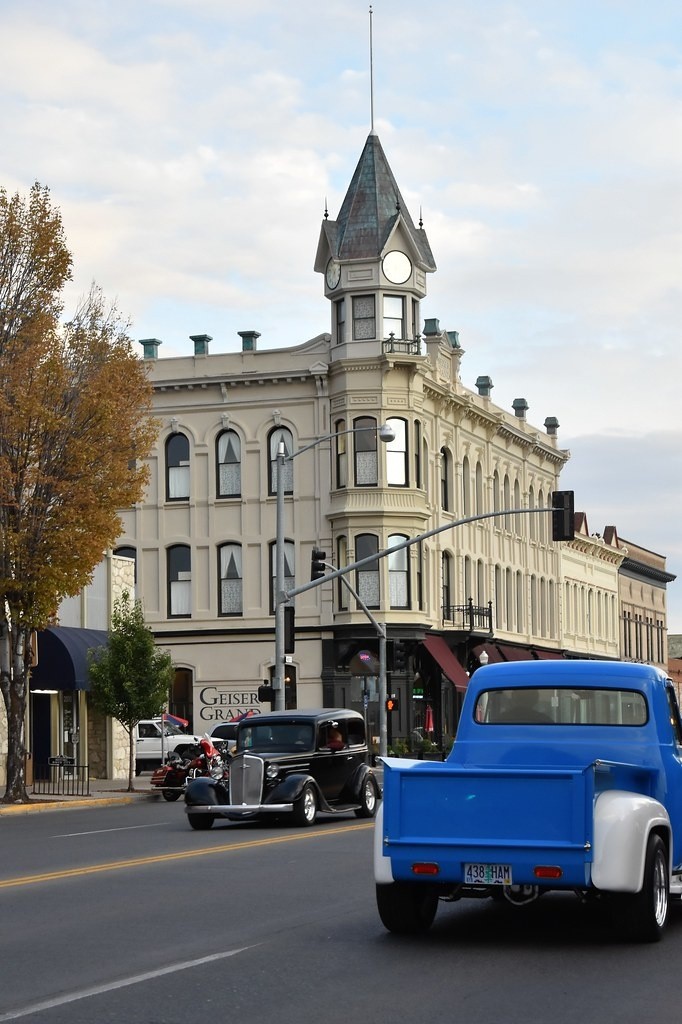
325,255,342,290
382,249,411,285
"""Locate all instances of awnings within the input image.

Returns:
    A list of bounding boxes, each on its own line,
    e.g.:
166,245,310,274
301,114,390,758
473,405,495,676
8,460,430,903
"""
421,632,566,695
28,625,136,690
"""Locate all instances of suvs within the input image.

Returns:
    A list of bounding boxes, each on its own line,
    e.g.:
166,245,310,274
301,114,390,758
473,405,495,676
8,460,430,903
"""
135,719,229,777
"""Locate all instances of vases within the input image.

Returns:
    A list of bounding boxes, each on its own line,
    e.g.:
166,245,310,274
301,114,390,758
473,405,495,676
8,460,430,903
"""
421,752,443,761
372,754,394,767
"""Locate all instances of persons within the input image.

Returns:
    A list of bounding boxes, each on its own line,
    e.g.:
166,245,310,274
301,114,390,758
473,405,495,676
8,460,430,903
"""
301,724,346,752
494,688,556,724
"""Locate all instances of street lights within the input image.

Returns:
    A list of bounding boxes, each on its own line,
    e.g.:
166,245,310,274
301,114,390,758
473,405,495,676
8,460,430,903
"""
272,425,397,716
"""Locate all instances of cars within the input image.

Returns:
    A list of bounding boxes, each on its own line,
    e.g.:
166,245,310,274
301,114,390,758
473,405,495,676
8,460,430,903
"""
209,721,273,754
184,708,383,831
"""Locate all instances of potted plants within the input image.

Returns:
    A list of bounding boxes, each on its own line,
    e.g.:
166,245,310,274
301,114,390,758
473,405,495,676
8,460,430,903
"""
372,745,395,755
443,738,453,760
394,738,418,759
422,741,442,752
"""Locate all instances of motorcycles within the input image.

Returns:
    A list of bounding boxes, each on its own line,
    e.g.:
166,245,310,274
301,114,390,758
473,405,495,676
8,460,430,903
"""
150,733,232,802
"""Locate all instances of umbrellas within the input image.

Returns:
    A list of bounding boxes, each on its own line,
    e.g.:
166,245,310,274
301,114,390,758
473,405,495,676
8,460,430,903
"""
229,711,259,723
156,714,188,728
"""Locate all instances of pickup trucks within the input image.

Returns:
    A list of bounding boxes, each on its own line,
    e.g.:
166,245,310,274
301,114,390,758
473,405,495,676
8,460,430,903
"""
374,658,682,945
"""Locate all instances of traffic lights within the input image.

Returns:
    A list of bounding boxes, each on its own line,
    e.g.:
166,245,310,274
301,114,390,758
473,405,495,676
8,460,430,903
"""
386,697,399,712
309,548,327,583
